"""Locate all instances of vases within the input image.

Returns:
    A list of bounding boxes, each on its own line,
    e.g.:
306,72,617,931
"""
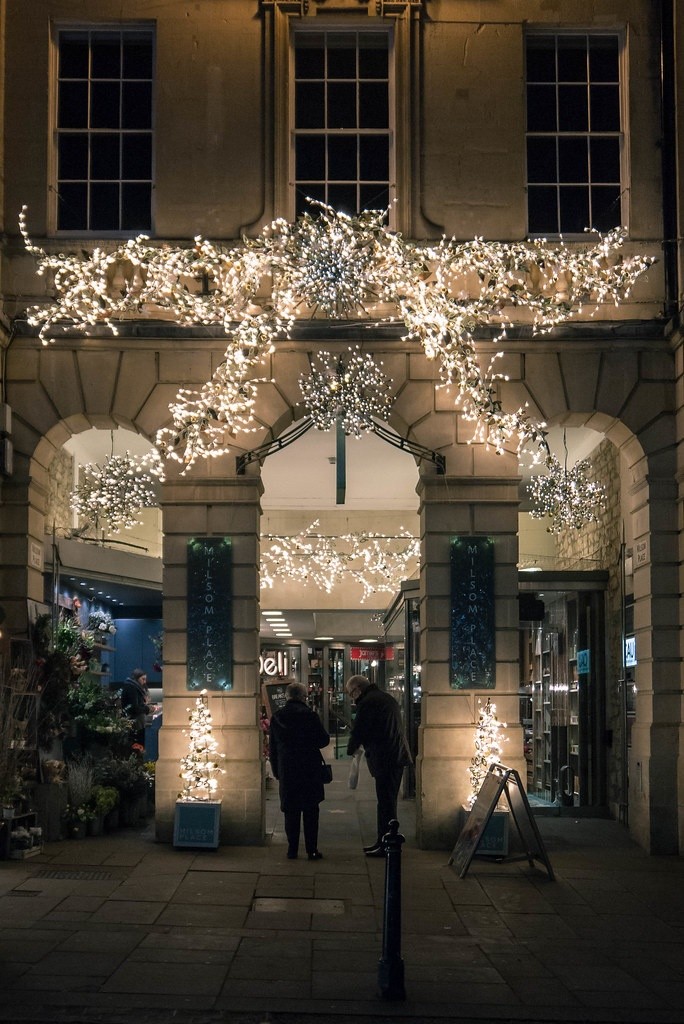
71,794,149,839
11,845,44,860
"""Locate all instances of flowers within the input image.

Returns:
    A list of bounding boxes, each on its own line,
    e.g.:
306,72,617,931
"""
65,744,155,816
36,610,139,754
9,827,44,850
66,802,98,832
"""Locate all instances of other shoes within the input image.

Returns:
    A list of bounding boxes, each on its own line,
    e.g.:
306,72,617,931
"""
287,853,298,859
308,852,322,861
363,839,387,857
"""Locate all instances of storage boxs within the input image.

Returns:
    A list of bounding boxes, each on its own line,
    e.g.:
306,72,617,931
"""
0,812,38,860
0,637,44,782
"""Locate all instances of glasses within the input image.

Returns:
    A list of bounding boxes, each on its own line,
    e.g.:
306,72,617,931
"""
349,685,361,698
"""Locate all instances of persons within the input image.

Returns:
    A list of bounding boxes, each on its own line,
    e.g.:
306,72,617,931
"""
344,673,414,858
270,682,330,860
121,668,155,763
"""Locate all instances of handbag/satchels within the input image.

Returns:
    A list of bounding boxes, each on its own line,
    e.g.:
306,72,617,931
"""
348,749,364,790
321,764,333,784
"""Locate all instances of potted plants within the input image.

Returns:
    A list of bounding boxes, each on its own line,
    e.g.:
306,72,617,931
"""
0,781,23,818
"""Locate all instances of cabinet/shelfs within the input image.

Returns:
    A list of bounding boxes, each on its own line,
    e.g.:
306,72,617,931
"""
520,623,584,808
87,646,115,676
